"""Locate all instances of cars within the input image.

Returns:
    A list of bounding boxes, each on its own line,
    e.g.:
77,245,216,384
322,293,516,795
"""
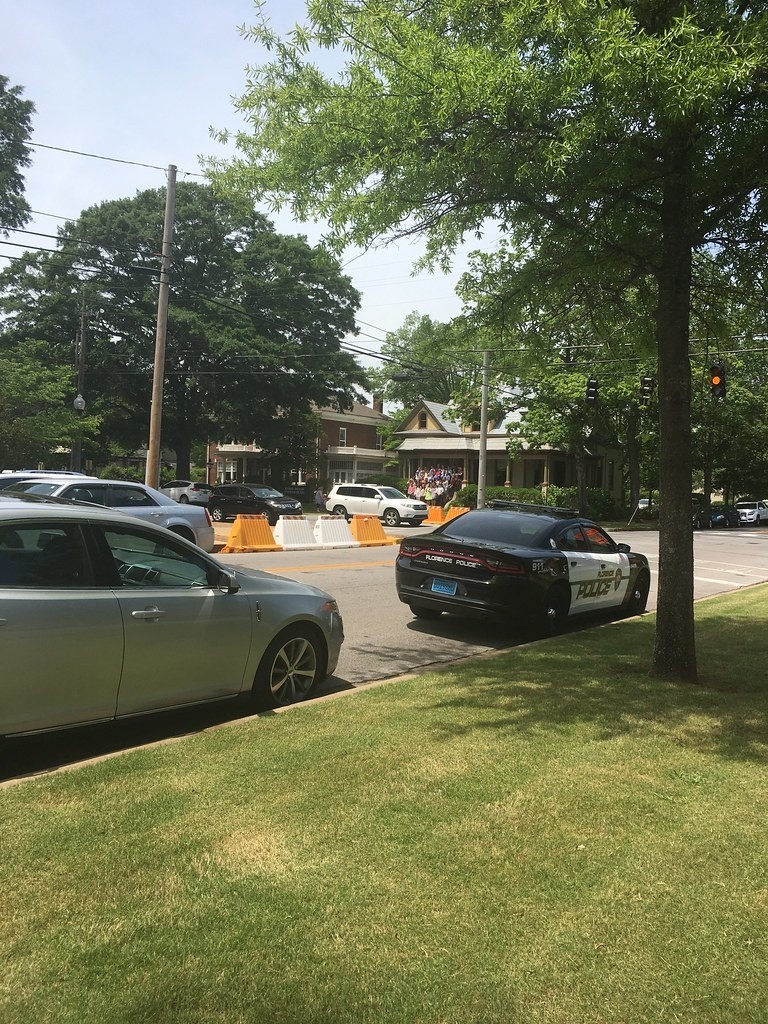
638,492,768,530
2,479,215,555
0,490,346,740
395,497,650,635
159,478,214,506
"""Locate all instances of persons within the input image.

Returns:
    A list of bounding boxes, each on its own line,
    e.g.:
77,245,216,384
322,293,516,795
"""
315,486,323,513
408,464,463,506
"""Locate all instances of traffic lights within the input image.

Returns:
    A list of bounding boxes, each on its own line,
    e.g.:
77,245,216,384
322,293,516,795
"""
639,376,654,407
584,376,599,404
708,364,727,398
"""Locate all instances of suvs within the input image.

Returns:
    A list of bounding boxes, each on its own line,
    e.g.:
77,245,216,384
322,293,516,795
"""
0,469,100,491
207,481,304,524
323,483,430,529
